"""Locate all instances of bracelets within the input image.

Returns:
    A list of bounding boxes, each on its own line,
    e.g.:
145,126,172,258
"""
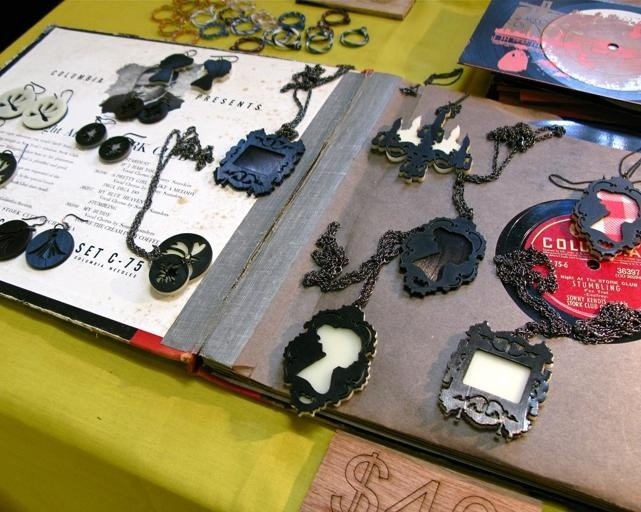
151,0,369,54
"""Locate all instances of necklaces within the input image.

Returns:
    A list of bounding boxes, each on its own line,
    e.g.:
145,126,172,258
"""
401,122,565,297
550,148,641,259
128,126,213,295
438,248,641,439
215,65,355,197
284,221,406,417
370,92,473,185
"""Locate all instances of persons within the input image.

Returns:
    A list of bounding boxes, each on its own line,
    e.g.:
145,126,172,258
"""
103,68,184,123
